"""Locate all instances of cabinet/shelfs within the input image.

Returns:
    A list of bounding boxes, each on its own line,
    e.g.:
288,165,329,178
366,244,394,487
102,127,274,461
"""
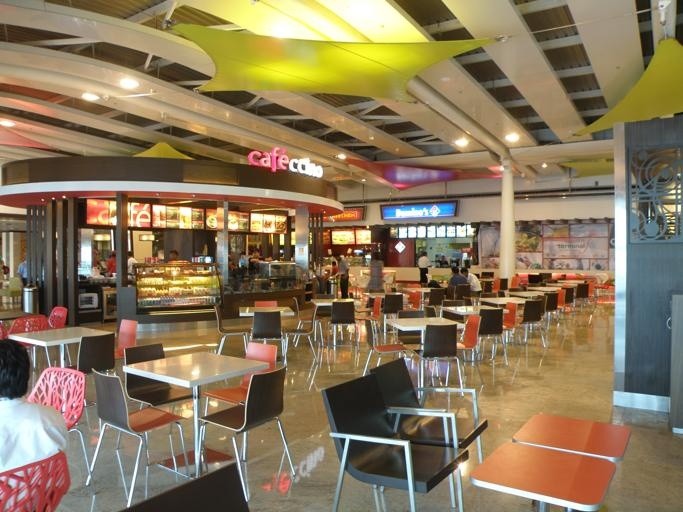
134,263,223,312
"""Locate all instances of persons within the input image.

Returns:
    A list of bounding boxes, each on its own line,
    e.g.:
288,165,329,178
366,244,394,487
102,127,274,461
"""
365,252,385,292
247,248,272,273
107,250,116,278
417,252,431,283
440,255,448,265
126,251,138,275
459,268,481,297
331,261,338,276
17,255,27,311
448,266,467,299
167,250,178,261
333,254,350,299
0,337,67,512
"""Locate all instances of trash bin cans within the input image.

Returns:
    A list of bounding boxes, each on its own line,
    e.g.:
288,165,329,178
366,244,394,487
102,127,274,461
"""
22,284,40,314
327,276,338,299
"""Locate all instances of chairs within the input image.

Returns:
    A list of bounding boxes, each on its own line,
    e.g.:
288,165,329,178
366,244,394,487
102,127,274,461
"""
366,358,488,508
0,451,71,512
118,463,251,512
285,295,334,348
417,279,476,397
327,301,359,349
113,320,138,361
0,305,71,368
361,283,417,374
213,305,249,357
23,367,92,488
245,312,288,370
320,372,469,512
116,343,196,461
557,272,608,312
84,368,191,509
475,272,557,343
75,333,116,406
196,366,296,501
254,301,278,308
205,342,277,458
